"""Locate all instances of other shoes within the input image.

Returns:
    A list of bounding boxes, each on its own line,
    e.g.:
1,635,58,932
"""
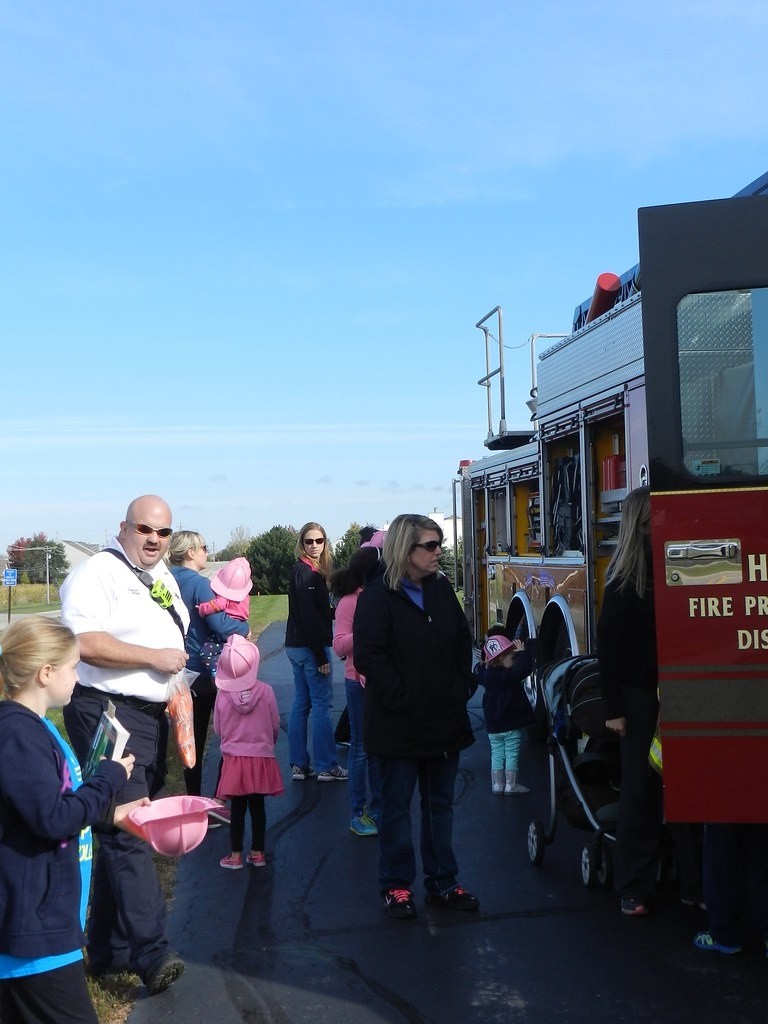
145,954,185,992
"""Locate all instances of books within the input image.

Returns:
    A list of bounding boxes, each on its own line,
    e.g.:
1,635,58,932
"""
81,710,131,825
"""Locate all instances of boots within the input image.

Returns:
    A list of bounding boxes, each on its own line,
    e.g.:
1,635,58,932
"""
504,770,529,795
491,769,505,795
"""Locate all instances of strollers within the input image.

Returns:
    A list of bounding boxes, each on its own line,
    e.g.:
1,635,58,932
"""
527,653,706,891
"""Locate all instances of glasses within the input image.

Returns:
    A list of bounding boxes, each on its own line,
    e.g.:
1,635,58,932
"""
412,541,441,553
188,545,208,554
303,538,325,545
125,518,172,539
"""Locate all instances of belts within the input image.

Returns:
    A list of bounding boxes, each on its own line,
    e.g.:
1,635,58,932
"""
79,687,166,718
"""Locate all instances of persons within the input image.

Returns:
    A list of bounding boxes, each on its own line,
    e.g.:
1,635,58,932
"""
590,484,768,955
195,557,254,622
164,529,252,826
325,512,477,918
211,634,280,870
0,614,151,1024
472,634,532,794
284,523,349,781
59,495,191,995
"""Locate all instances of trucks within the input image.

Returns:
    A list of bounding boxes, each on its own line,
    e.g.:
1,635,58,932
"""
451,172,768,862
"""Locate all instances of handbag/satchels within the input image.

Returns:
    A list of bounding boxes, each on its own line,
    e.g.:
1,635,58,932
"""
168,670,196,769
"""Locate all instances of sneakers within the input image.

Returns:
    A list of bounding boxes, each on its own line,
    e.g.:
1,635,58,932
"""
207,819,223,829
384,886,416,918
317,764,350,780
693,929,742,952
350,812,379,835
293,765,316,780
425,884,479,911
207,798,232,824
621,894,648,916
246,852,265,867
220,851,243,869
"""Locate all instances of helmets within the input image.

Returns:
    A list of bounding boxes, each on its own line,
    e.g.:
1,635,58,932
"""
214,634,260,693
130,794,223,859
482,636,514,661
209,557,254,601
361,530,389,549
